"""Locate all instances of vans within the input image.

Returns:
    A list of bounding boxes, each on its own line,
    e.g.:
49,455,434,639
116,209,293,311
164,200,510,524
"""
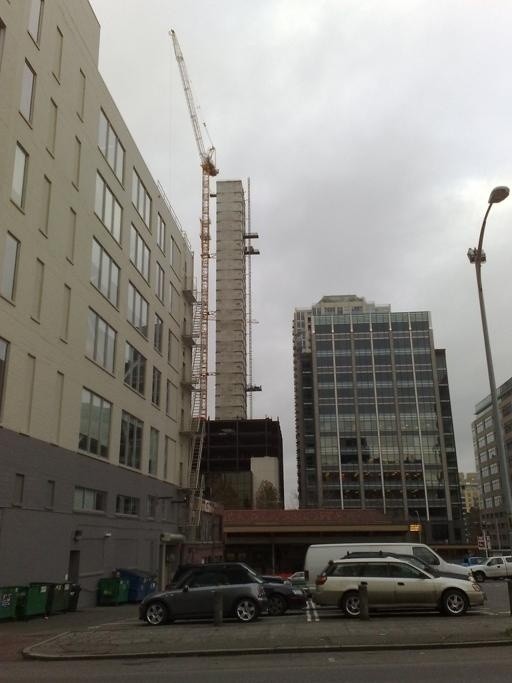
303,541,478,595
468,555,511,583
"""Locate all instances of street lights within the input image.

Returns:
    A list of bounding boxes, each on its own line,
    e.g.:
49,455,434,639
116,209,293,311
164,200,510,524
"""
467,184,511,535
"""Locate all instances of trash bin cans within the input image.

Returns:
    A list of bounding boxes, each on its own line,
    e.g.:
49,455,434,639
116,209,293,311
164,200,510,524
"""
98,568,157,606
0,582,81,620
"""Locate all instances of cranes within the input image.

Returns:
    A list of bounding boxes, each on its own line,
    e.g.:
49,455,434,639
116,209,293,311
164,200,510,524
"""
167,28,220,424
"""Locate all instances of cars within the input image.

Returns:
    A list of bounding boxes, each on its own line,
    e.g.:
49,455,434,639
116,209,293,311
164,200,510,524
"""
138,560,306,627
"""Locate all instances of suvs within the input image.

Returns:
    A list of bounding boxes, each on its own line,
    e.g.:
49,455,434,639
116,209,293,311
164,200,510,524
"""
312,551,486,620
